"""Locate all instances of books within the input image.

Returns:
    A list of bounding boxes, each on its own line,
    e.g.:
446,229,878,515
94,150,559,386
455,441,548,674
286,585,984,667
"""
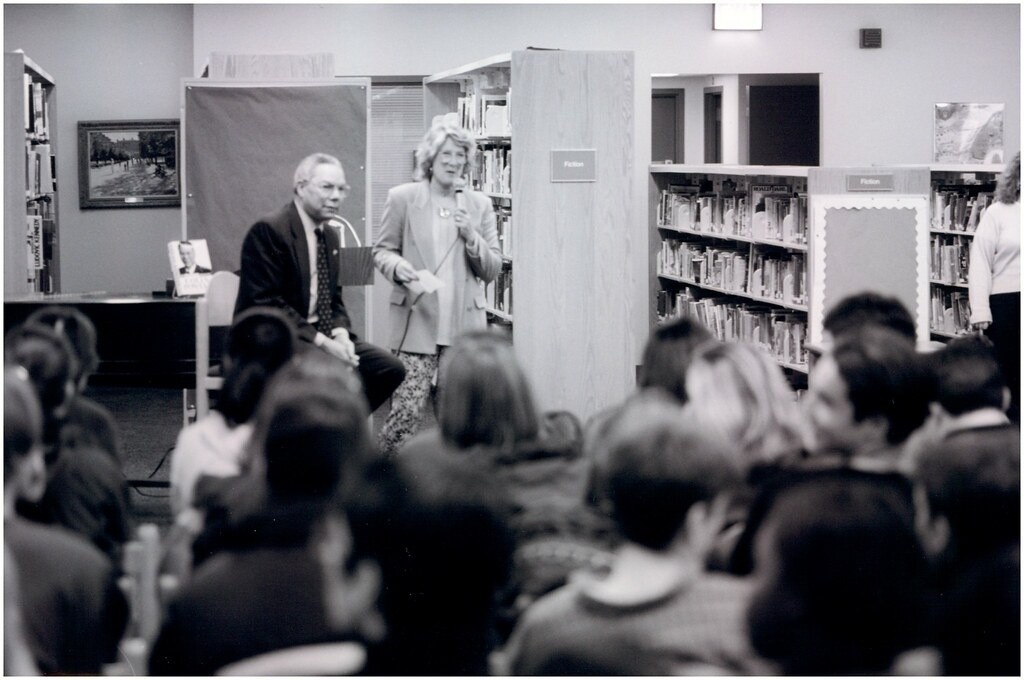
167,239,213,296
650,176,809,246
651,231,804,304
23,69,58,298
455,89,515,319
650,286,807,369
928,184,992,334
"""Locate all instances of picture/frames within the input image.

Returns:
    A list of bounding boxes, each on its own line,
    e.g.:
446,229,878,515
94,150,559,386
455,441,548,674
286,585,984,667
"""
77,117,182,211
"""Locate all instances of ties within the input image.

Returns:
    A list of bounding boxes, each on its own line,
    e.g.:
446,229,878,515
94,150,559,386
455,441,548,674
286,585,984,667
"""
314,227,333,337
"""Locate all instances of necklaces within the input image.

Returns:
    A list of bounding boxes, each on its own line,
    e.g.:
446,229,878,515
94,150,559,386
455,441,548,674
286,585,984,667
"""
432,189,456,219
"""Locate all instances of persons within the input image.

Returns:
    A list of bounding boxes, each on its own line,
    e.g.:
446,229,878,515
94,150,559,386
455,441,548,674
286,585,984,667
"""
177,238,215,274
3,307,138,676
373,120,502,462
964,151,1021,427
134,315,718,677
227,155,405,422
685,289,1020,677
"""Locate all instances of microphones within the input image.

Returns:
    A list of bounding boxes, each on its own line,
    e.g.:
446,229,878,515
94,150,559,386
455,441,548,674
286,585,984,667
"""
453,177,466,211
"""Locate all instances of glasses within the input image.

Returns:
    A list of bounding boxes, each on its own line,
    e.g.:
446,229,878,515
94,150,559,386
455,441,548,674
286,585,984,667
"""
308,180,350,198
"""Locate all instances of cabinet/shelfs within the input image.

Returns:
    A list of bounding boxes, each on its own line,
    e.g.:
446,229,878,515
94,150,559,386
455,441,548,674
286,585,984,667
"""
421,50,643,425
651,163,934,392
903,162,1006,340
3,52,63,299
